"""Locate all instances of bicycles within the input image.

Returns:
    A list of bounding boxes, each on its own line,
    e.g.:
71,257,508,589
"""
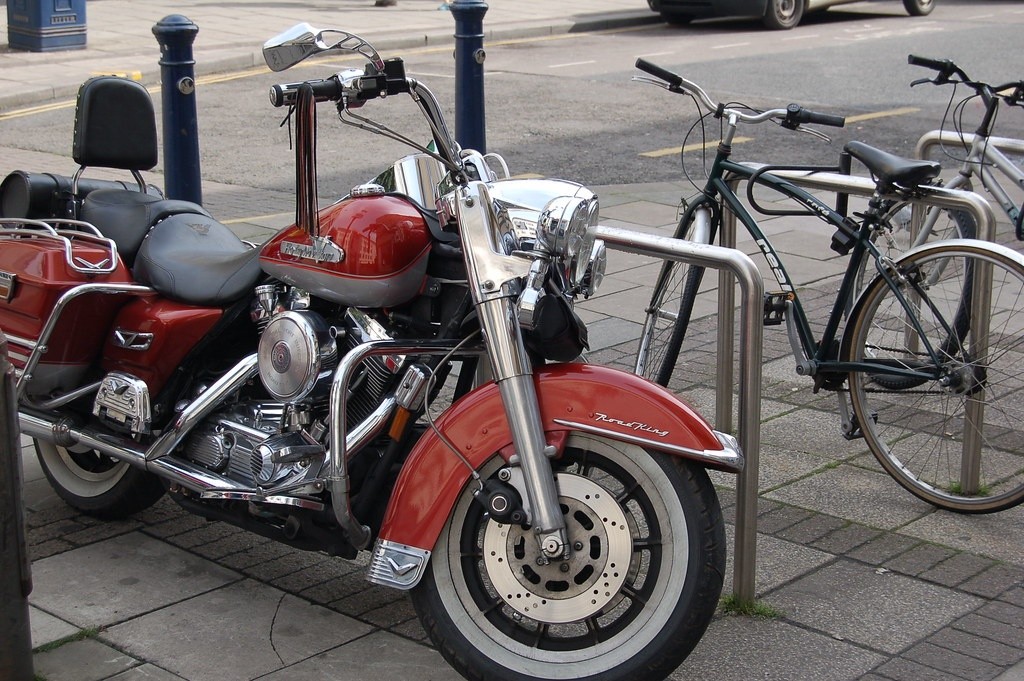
840,54,1024,389
631,59,1024,517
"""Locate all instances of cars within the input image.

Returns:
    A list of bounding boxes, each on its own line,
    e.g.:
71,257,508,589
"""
645,0,936,31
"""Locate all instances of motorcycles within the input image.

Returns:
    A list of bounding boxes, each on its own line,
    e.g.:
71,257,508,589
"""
0,22,745,681
